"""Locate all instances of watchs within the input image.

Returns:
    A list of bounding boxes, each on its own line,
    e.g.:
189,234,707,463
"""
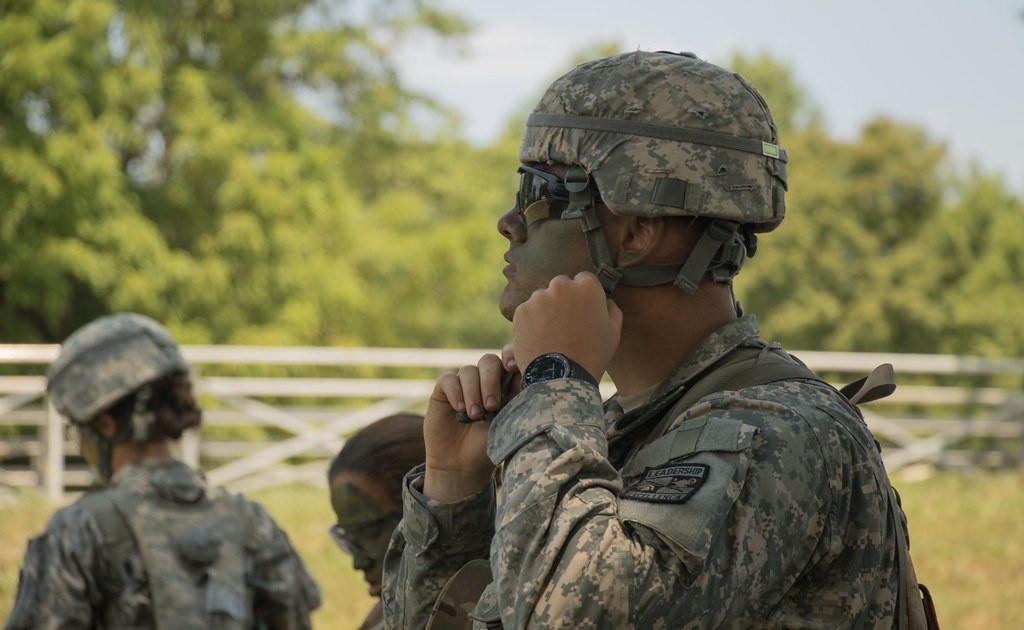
521,352,599,389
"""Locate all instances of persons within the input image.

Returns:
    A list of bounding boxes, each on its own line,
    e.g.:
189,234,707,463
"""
327,411,426,630
381,50,940,630
5,312,323,630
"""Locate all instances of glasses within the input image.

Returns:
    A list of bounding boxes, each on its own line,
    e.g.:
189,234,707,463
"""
328,511,403,553
515,165,604,225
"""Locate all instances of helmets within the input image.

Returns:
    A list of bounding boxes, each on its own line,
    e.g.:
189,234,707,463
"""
44,314,191,424
520,50,788,235
